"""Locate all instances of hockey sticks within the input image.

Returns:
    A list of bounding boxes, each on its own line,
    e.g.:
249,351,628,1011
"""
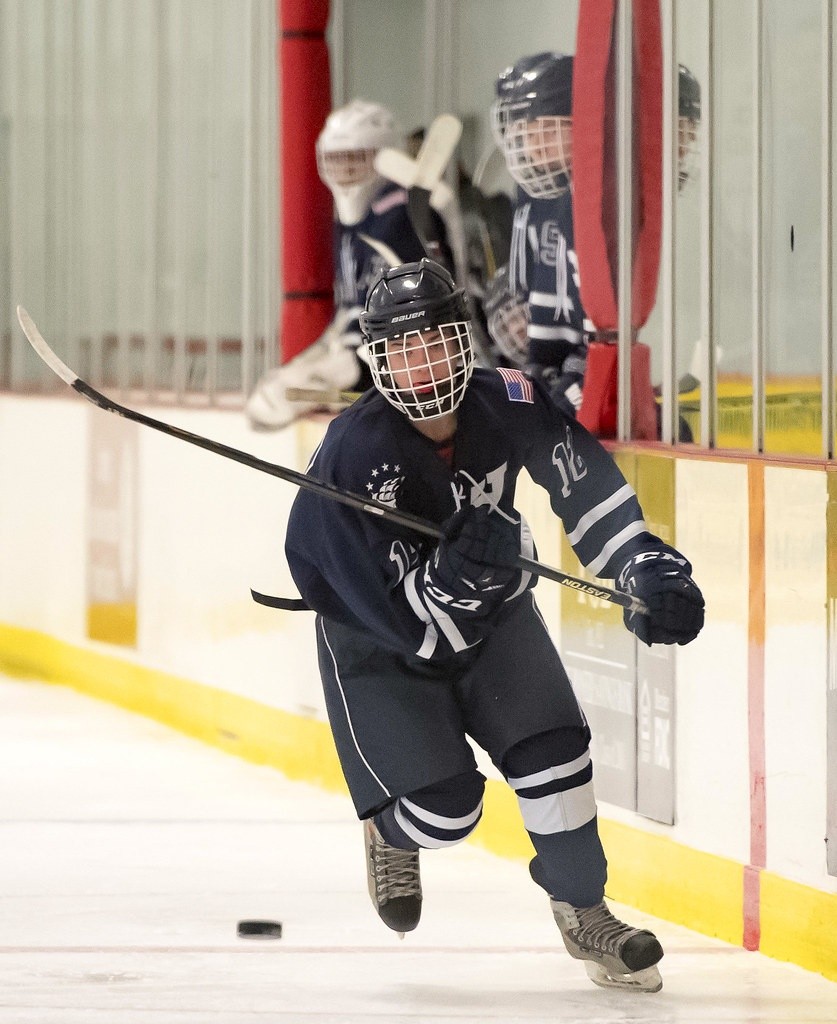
403,115,464,275
369,145,469,302
15,302,648,614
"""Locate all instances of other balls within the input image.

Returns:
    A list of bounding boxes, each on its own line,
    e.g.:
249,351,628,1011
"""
237,918,284,942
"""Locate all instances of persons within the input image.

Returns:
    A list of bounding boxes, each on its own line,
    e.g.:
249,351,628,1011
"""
246,51,700,441
284,258,706,994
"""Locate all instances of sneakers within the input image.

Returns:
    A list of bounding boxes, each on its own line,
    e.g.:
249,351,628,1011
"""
550,896,663,993
363,813,421,939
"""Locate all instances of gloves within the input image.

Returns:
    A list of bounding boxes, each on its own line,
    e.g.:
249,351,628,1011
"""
434,505,520,601
615,546,706,646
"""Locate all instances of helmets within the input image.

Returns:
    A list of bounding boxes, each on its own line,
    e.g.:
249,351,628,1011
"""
490,52,574,201
357,258,472,421
481,262,535,365
678,64,702,200
316,99,399,225
